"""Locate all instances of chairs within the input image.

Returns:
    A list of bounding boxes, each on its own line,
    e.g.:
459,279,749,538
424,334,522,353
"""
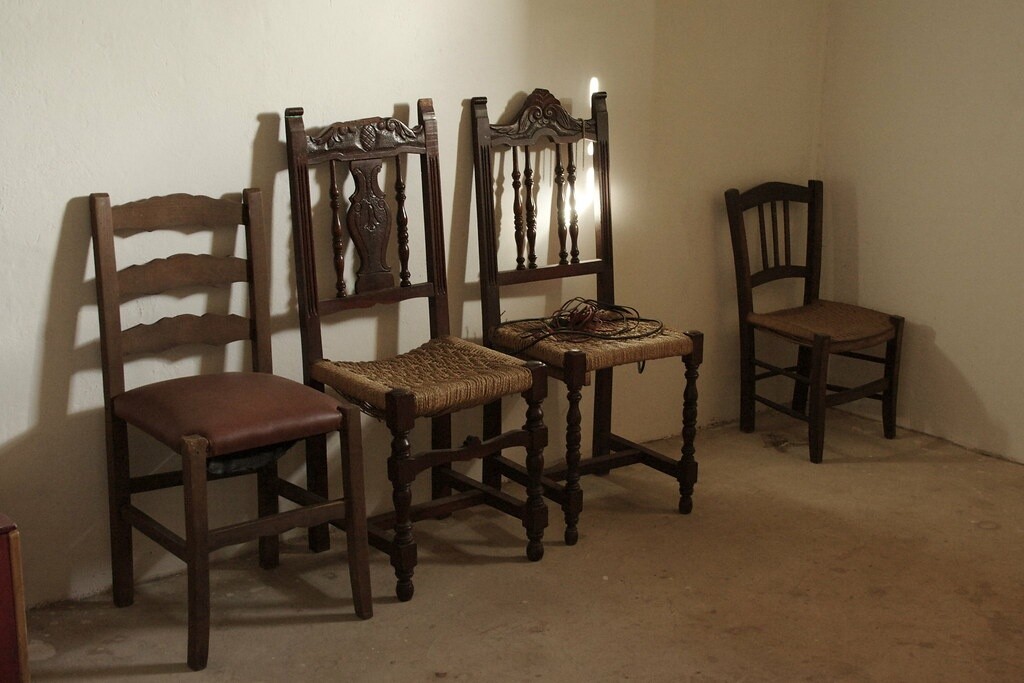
724,180,906,463
285,98,548,602
88,188,374,671
471,88,705,546
